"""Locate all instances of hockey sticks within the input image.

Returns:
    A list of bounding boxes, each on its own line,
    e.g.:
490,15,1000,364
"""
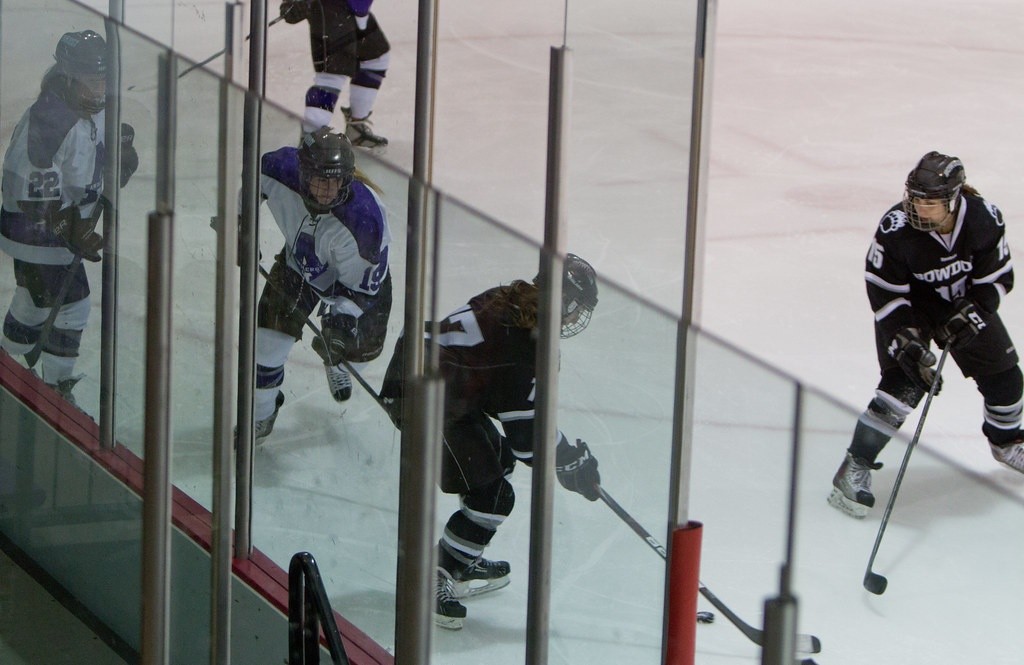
594,480,821,656
862,335,955,596
127,15,283,93
258,262,386,413
6,189,106,369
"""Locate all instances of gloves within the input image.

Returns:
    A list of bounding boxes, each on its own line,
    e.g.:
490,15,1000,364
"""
120,123,138,188
47,205,103,262
556,439,600,502
280,0,311,24
324,314,357,363
935,298,987,350
886,329,943,396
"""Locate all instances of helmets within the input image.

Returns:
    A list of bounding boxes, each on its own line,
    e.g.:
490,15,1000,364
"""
296,126,355,208
904,152,965,215
55,30,111,117
533,254,600,340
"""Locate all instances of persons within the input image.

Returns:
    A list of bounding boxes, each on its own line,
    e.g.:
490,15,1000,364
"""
0,29,139,425
378,249,600,629
827,152,1023,518
280,0,392,155
233,133,393,453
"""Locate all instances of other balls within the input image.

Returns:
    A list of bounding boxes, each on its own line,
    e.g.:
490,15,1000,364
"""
697,611,714,623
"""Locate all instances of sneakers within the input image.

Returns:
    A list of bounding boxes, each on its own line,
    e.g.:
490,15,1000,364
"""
234,391,284,450
989,443,1023,475
326,364,353,402
44,373,95,420
828,452,875,519
434,574,467,629
341,107,389,147
434,539,511,598
